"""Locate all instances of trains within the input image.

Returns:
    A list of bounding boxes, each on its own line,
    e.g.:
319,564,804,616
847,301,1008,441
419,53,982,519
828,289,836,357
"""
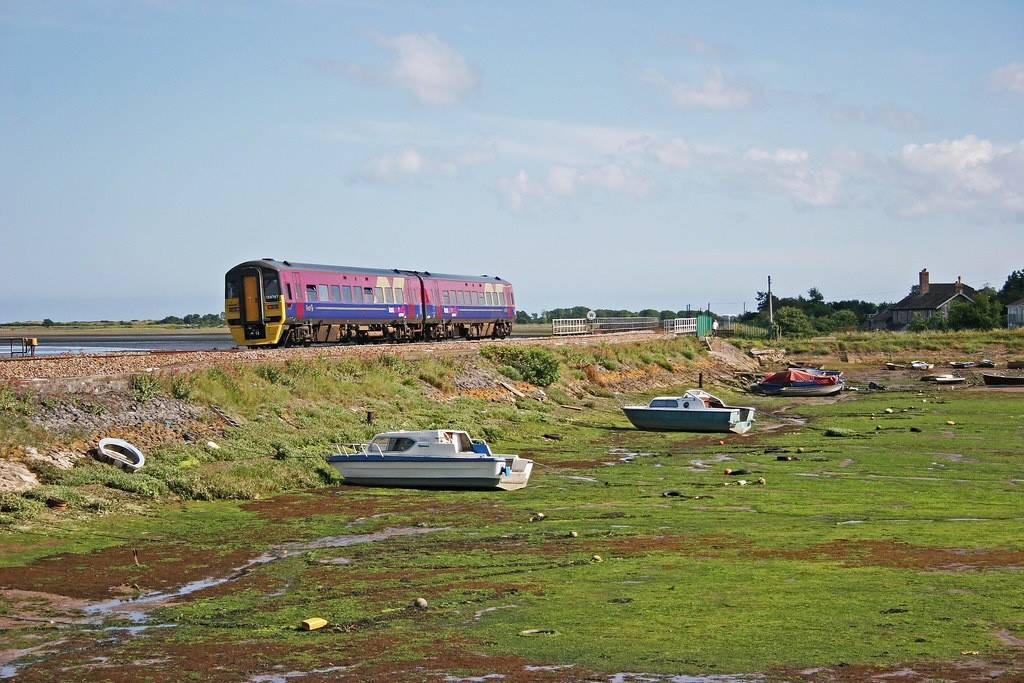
221,256,515,347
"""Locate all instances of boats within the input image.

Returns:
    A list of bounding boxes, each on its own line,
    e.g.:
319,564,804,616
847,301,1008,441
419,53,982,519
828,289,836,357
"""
936,376,966,386
885,360,935,371
619,387,755,438
982,374,1024,388
948,359,975,368
753,356,844,396
324,426,533,493
1008,362,1024,369
